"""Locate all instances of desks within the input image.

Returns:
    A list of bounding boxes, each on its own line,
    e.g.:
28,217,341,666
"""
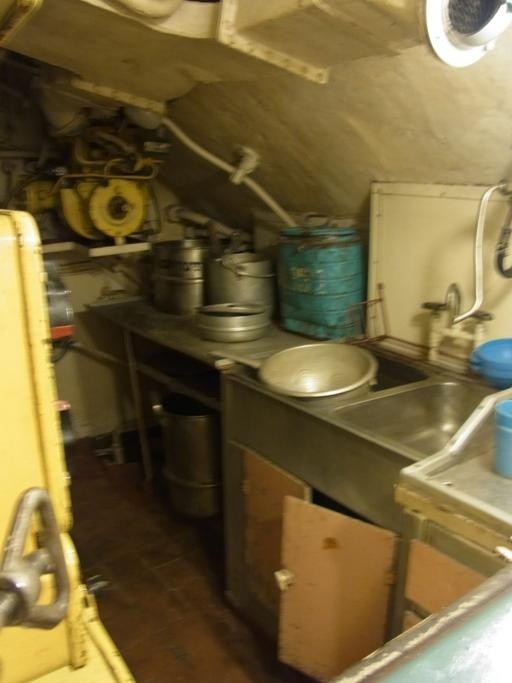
83,299,325,485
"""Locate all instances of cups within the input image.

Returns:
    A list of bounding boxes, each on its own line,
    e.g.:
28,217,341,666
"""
493,400,511,479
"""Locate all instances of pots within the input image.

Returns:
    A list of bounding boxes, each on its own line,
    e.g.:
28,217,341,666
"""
152,273,207,313
152,239,210,279
211,343,379,405
208,252,278,304
195,303,270,342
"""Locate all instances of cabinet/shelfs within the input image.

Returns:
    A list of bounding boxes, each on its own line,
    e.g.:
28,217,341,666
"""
219,440,511,683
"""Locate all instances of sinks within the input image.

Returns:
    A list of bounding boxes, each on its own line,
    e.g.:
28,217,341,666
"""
230,340,434,405
331,379,497,469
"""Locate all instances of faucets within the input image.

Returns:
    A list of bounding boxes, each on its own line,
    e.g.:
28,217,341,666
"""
452,180,504,326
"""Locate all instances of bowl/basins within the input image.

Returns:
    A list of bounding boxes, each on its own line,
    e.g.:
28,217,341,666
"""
469,336,512,388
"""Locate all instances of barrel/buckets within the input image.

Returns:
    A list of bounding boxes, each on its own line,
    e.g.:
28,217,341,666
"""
207,244,276,314
153,237,211,314
275,212,369,339
